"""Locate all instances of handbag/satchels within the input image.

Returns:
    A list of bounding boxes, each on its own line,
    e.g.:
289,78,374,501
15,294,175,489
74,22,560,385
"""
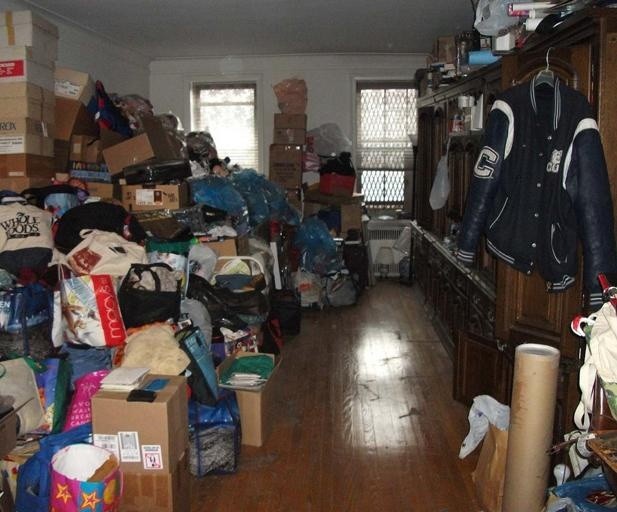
13,422,123,512
188,388,242,478
0,357,73,435
58,261,129,347
171,325,220,407
118,263,182,329
64,369,112,432
0,202,54,268
64,228,147,277
0,284,63,360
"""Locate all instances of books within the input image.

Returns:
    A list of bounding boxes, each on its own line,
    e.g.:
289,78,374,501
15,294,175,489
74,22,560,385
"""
218,352,277,389
96,365,153,392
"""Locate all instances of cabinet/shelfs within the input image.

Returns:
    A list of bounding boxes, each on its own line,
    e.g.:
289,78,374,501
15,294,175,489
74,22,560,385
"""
409,19,614,422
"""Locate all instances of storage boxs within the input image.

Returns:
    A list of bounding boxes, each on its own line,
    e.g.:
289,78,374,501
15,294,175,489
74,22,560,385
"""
52,95,95,148
66,347,284,512
55,69,96,107
274,113,305,144
269,143,300,189
136,211,183,239
69,135,101,164
273,78,308,113
1,117,53,158
1,8,58,59
103,132,175,176
2,179,52,194
202,237,237,273
149,234,190,256
319,173,355,197
302,187,363,238
1,82,56,124
100,128,125,162
2,45,56,93
287,191,301,211
69,162,112,183
119,180,186,214
78,180,113,200
1,154,51,179
